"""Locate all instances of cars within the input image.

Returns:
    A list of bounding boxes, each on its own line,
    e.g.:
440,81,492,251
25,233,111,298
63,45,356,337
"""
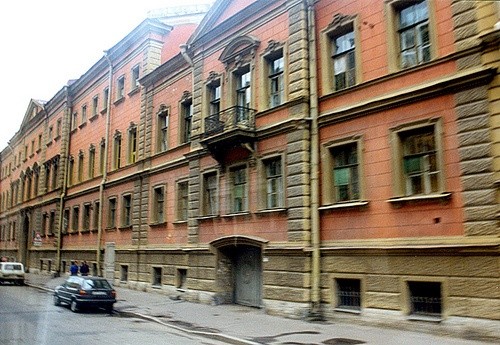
53,276,116,313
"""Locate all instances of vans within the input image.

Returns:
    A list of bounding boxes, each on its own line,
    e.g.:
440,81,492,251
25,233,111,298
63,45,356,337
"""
0,262,25,286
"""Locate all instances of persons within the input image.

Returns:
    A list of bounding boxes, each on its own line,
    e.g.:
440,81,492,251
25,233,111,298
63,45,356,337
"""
80,261,89,276
69,261,78,276
